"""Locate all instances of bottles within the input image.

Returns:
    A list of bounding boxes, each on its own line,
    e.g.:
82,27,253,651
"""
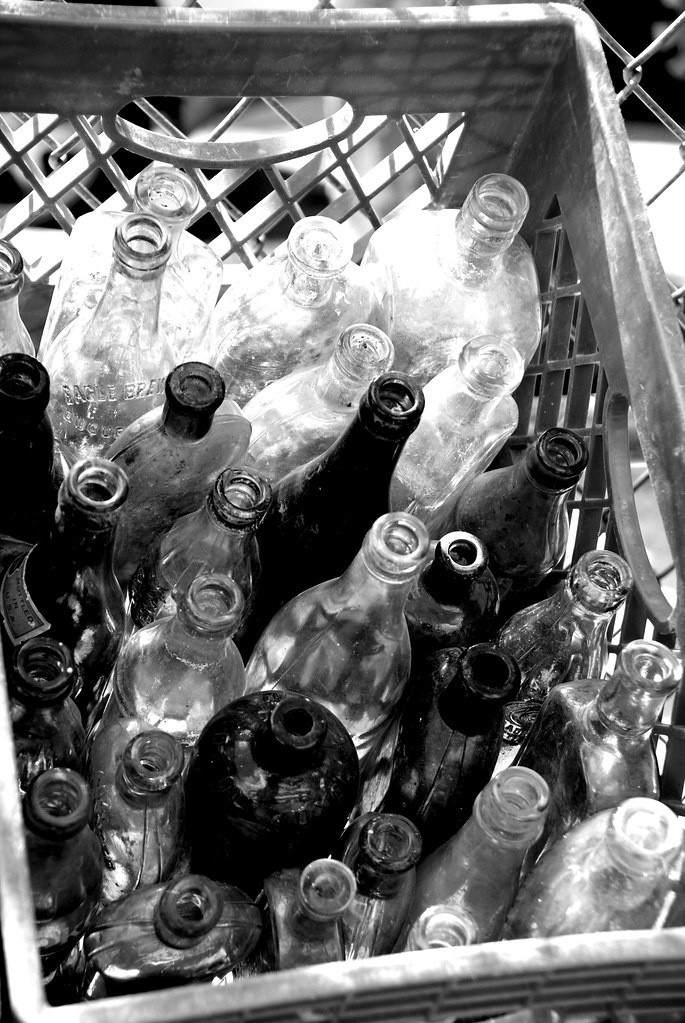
0,156,685,1000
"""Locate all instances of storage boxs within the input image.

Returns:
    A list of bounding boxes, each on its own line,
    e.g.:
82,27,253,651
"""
0,1,685,1023
